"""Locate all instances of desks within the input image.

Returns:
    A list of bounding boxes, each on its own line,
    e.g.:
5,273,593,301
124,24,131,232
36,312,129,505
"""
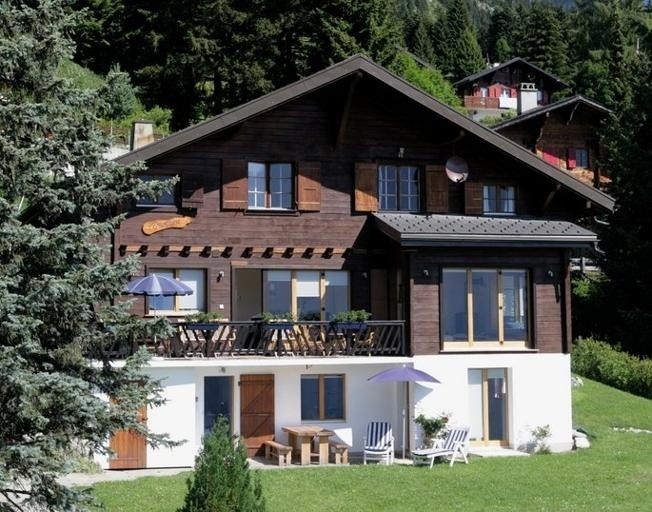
281,425,335,466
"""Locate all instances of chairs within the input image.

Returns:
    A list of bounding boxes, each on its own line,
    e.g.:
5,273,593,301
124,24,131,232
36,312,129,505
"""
363,421,394,466
264,317,375,358
411,428,470,467
177,315,237,357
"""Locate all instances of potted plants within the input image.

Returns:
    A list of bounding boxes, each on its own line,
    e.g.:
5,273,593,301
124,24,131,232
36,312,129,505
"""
417,412,450,447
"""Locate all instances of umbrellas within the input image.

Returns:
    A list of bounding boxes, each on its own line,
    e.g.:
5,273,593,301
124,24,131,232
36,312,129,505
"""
366,362,442,459
118,272,194,343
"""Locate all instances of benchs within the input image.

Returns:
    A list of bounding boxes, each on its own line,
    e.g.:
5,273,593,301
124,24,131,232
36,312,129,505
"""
263,438,294,467
329,440,349,465
